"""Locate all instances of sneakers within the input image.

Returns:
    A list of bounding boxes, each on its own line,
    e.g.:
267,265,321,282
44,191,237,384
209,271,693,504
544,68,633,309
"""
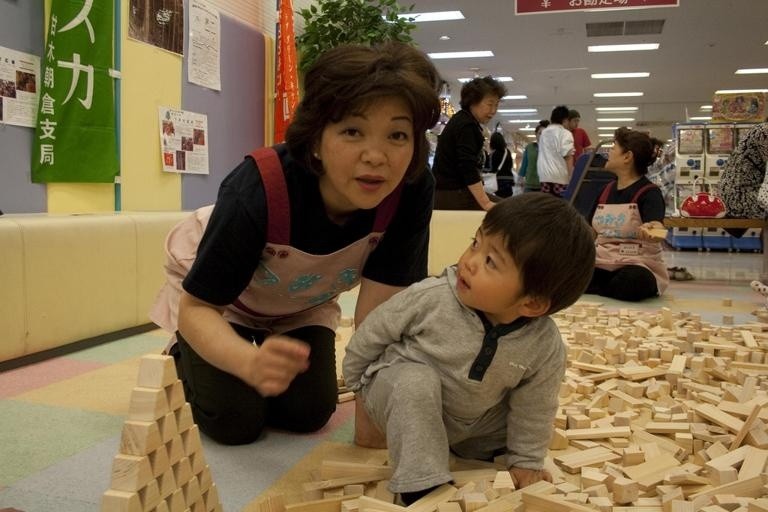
666,266,693,281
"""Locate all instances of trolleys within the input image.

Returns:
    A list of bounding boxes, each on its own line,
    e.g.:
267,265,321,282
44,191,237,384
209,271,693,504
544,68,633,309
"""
559,138,616,209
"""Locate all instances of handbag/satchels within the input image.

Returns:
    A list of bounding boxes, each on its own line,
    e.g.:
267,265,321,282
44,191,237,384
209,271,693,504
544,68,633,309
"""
679,177,727,219
479,172,499,194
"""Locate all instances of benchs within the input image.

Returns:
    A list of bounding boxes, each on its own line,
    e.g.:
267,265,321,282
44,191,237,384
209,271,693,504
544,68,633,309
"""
428,209,487,277
0,210,195,374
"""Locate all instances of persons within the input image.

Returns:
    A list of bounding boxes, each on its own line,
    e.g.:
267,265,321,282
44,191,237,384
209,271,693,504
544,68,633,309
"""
433,76,667,302
714,116,768,299
338,189,597,506
145,40,450,446
